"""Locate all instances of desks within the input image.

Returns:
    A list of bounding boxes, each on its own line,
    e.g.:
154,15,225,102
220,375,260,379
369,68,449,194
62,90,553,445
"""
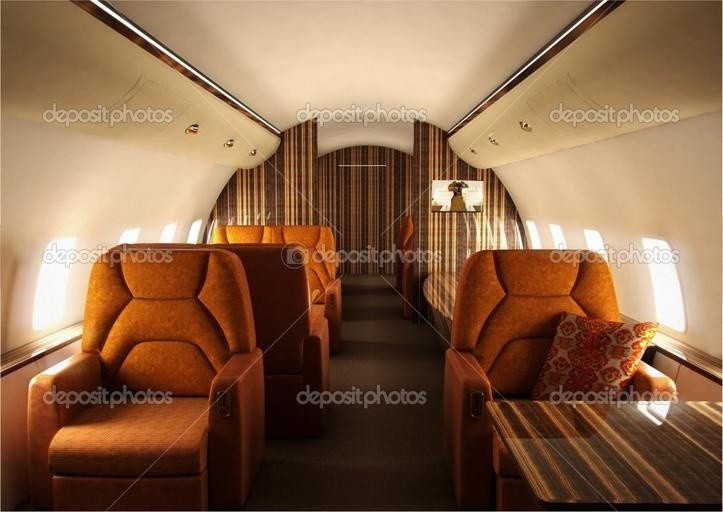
484,399,722,510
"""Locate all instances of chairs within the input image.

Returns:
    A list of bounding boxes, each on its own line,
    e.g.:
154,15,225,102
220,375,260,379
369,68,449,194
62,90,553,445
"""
442,250,677,510
402,233,414,319
397,216,414,291
152,245,330,439
27,243,265,512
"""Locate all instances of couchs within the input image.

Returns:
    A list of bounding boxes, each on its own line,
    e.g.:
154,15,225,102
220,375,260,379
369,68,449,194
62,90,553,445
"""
210,224,342,355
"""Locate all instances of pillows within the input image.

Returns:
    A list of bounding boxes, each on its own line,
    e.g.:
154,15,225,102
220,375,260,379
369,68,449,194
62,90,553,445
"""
529,311,661,401
